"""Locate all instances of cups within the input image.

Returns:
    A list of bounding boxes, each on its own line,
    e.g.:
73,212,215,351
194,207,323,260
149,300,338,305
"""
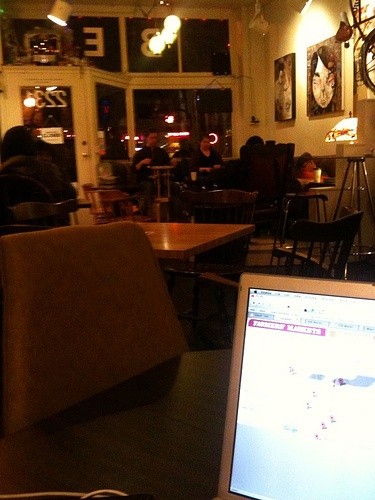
191,171,198,181
312,168,322,183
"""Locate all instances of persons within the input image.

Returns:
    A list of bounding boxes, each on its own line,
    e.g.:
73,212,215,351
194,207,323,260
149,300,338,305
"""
132,128,305,234
0,126,77,235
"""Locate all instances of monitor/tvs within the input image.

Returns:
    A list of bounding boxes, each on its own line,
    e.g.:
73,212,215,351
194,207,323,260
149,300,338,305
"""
210,273,375,500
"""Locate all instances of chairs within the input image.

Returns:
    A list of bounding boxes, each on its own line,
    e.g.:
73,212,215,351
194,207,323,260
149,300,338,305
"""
0,166,364,440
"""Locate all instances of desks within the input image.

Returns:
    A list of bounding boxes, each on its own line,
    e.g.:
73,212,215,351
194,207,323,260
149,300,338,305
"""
135,222,257,332
0,347,231,500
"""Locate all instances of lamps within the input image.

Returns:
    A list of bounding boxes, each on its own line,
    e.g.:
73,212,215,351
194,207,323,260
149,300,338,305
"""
47,0,73,26
248,0,271,38
148,5,182,55
